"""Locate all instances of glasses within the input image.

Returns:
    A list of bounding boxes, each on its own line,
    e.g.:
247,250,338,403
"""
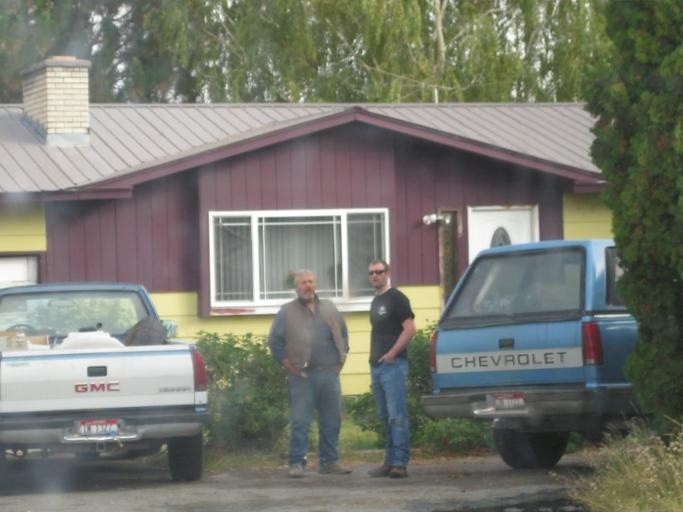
368,270,386,276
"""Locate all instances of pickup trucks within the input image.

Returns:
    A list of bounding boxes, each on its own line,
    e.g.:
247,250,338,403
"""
1,282,209,480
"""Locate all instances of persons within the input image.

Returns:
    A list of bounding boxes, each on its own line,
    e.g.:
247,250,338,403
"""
367,258,416,479
267,268,353,478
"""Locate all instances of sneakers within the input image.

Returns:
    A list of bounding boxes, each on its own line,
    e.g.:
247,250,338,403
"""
286,462,306,478
387,466,408,479
318,461,353,476
366,465,390,477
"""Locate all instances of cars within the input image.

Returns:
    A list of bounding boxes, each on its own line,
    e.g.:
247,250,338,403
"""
420,238,642,469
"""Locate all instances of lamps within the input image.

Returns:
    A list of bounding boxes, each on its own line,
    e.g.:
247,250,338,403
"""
420,212,453,226
